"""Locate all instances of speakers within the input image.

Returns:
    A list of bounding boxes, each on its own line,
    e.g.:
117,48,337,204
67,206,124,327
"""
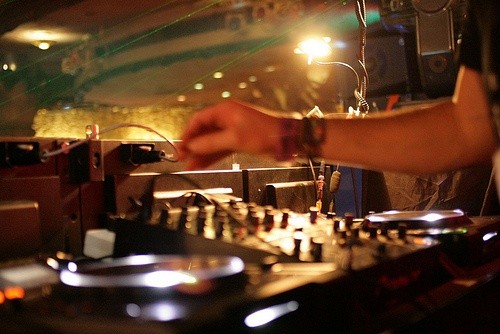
411,0,463,99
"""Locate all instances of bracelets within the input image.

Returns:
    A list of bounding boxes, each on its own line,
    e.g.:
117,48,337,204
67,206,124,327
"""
277,111,326,158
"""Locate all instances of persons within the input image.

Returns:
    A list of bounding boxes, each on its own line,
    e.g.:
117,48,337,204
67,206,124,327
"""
177,0,500,175
